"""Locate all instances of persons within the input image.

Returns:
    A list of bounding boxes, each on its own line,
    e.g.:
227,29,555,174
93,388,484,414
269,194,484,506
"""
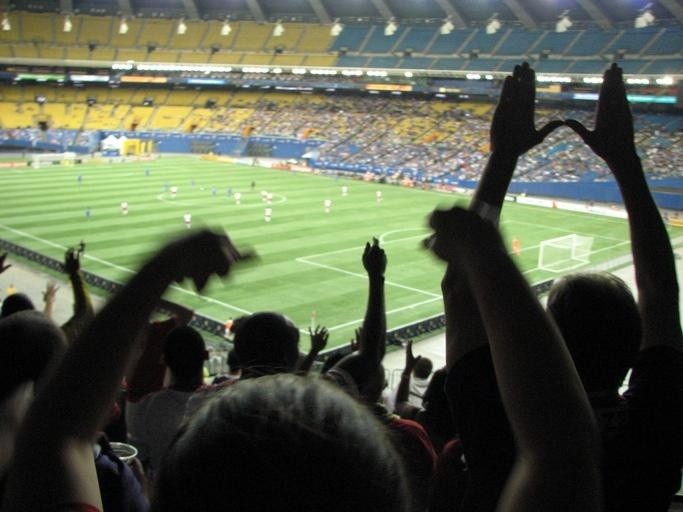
0,62,682,205
440,63,679,509
1,200,622,511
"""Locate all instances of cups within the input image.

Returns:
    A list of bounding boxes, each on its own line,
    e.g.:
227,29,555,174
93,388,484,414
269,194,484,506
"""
108,441,138,469
